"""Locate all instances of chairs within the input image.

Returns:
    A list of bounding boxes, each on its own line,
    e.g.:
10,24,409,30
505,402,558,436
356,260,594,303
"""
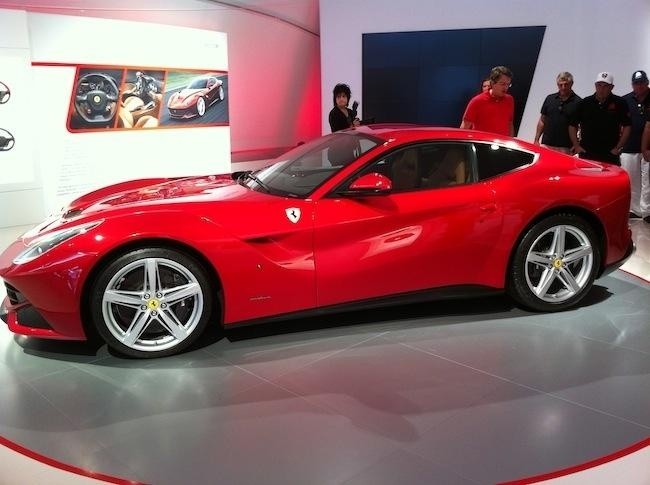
427,147,467,189
390,146,423,189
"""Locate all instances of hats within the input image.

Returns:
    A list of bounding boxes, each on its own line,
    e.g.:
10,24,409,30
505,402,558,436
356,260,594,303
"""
594,71,615,86
631,70,647,84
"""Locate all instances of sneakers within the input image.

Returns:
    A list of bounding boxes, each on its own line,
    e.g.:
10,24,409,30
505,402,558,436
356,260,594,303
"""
629,212,642,219
643,216,650,224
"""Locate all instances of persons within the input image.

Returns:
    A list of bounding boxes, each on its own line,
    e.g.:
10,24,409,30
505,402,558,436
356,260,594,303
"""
618,70,650,219
459,65,516,137
533,71,583,158
566,71,632,167
479,78,491,92
129,71,154,107
639,106,650,224
327,82,362,167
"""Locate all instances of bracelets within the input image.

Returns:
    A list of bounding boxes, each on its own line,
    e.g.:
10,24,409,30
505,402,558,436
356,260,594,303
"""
615,145,624,155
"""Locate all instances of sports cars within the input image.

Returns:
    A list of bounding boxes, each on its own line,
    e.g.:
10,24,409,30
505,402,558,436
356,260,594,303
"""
0,121,637,364
165,74,227,122
